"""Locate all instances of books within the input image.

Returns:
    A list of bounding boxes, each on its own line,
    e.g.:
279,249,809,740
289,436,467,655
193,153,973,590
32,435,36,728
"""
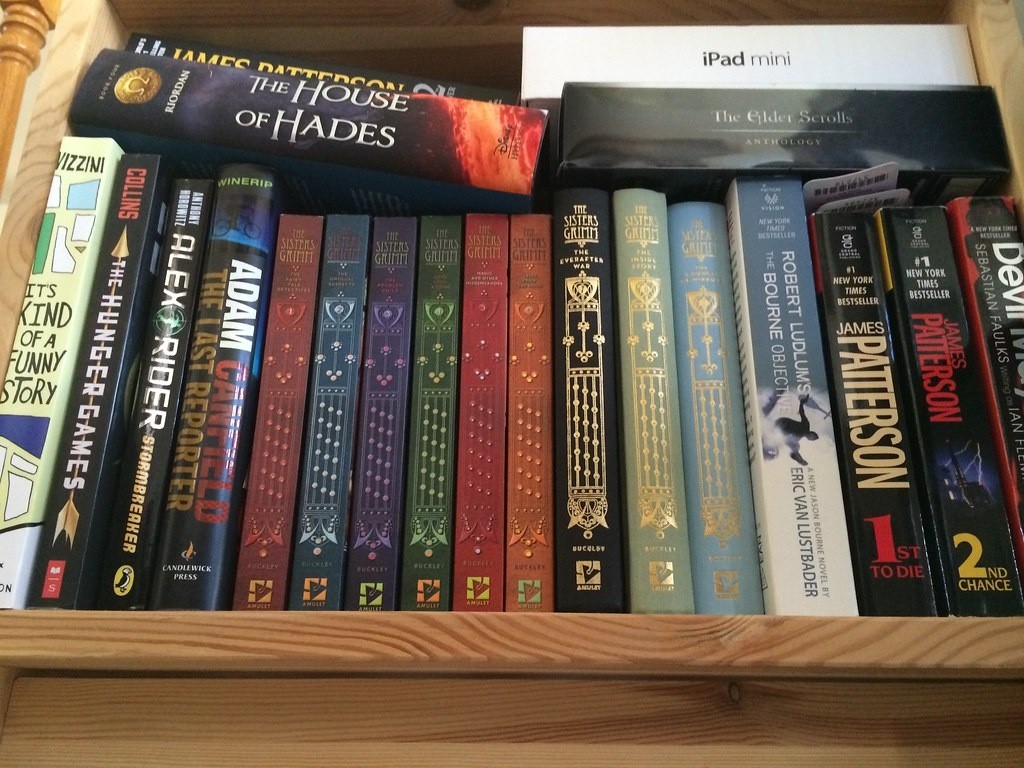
25,152,179,610
345,213,417,609
611,186,694,615
154,160,287,611
722,175,862,616
286,214,372,609
1,135,126,610
450,212,509,611
551,185,626,614
667,202,765,616
233,214,324,610
503,214,555,612
944,193,1024,598
399,215,461,612
68,31,549,212
94,179,214,611
872,206,1024,618
807,208,935,619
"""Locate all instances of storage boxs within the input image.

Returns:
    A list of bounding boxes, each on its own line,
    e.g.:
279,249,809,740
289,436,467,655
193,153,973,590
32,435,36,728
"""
520,23,978,110
547,81,1011,207
